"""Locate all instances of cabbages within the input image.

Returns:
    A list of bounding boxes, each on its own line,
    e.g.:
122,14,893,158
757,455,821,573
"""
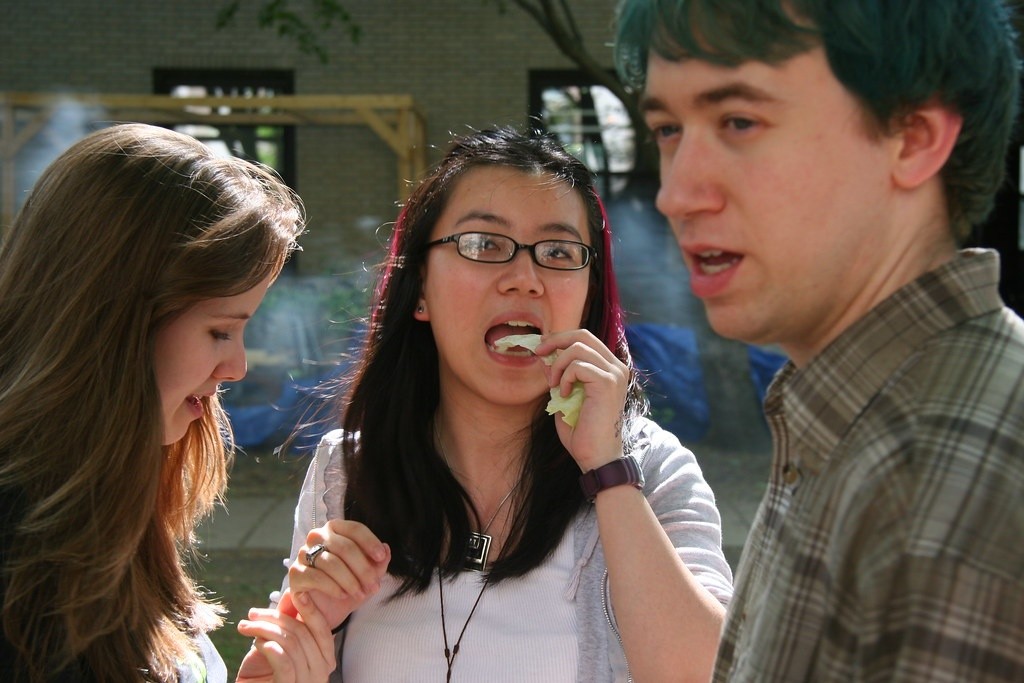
493,333,585,428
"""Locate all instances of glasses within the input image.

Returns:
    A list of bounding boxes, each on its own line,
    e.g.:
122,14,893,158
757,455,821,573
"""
426,232,601,271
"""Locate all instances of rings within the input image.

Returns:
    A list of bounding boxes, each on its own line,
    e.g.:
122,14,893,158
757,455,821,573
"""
304,543,328,569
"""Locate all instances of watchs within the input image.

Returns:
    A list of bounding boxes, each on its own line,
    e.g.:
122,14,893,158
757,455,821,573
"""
579,457,646,504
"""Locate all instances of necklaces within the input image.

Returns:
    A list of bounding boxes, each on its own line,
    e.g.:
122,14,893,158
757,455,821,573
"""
436,496,531,683
431,411,530,571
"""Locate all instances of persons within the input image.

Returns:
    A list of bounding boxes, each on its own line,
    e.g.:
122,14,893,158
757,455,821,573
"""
612,0,1024,682
0,122,337,683
248,129,734,683
